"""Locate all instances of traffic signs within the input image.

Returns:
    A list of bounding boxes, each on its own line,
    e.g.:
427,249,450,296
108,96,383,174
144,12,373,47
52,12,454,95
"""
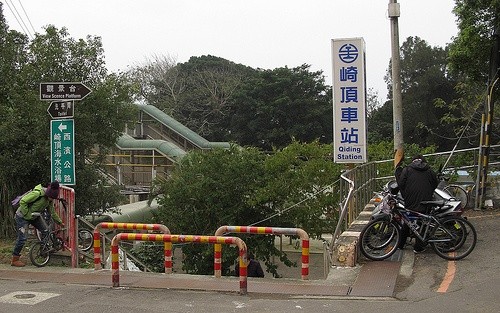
47,101,75,119
40,82,92,101
49,120,76,186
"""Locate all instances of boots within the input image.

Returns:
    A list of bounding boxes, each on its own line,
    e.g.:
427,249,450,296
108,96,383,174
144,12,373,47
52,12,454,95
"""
39,245,55,258
11,256,25,266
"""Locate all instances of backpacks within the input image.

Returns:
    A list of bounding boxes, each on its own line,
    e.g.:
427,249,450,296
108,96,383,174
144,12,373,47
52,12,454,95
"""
11,189,42,212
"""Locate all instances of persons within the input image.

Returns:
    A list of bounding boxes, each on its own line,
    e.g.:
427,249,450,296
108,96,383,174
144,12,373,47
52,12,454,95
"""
395,154,437,252
11,180,65,267
235,257,264,278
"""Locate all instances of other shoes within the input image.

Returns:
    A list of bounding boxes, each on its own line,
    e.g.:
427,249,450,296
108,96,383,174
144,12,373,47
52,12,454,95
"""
413,248,426,253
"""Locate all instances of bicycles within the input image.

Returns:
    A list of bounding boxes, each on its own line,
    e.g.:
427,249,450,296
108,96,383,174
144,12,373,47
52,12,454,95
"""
29,218,94,267
359,175,478,261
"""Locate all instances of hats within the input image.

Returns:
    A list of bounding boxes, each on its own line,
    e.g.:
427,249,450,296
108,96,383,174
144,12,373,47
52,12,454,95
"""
412,154,425,162
45,180,60,200
